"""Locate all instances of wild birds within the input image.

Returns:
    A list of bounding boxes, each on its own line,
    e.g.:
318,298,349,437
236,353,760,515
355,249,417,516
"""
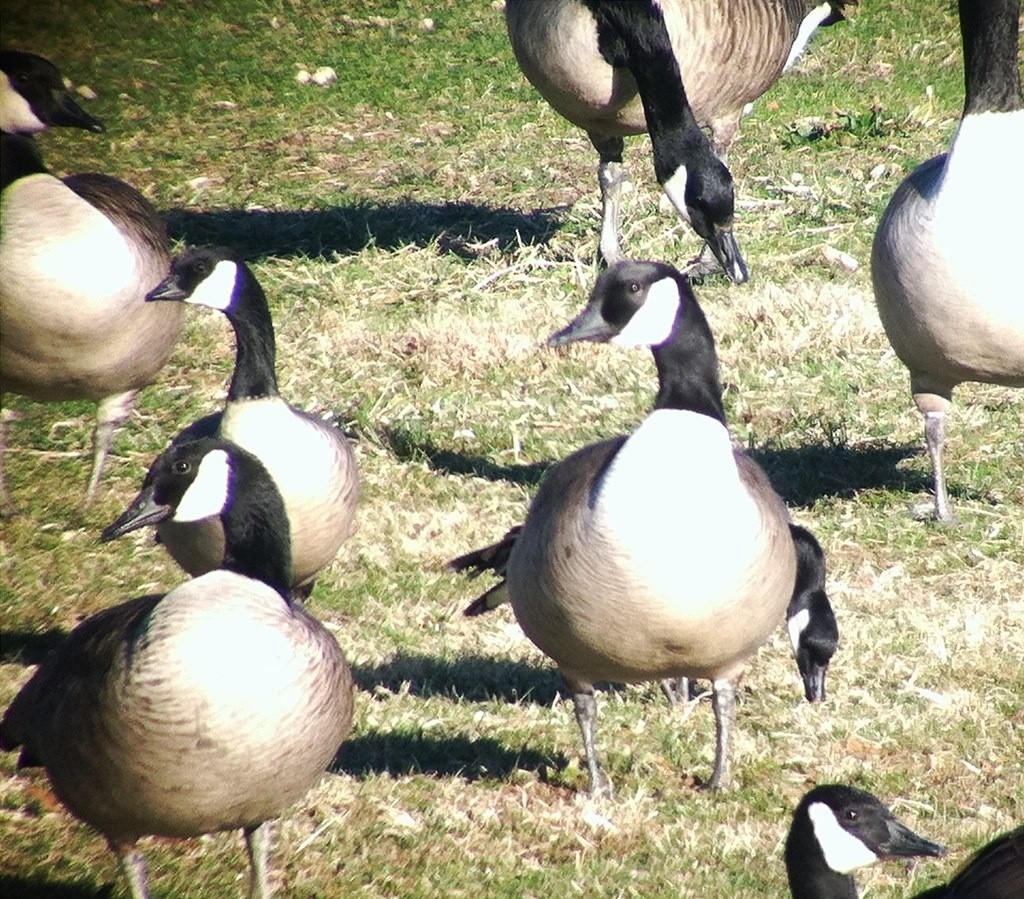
868,2,1024,538
505,259,802,811
0,46,179,510
98,246,354,603
500,1,848,286
1,435,357,899
783,785,1023,899
446,523,839,726
